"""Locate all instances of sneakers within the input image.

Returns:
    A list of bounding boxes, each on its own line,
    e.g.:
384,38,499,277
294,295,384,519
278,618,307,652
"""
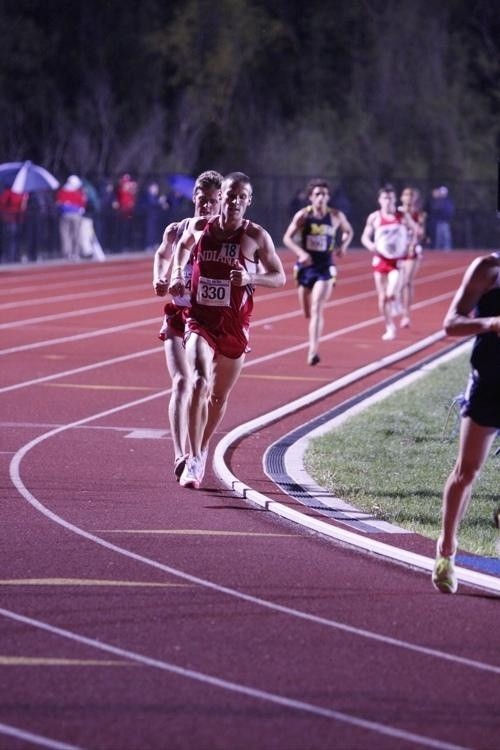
307,351,320,367
390,300,403,316
401,316,412,328
432,537,458,596
174,447,208,490
382,323,396,340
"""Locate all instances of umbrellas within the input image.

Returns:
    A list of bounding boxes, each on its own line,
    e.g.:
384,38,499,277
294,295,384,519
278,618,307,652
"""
1,160,61,195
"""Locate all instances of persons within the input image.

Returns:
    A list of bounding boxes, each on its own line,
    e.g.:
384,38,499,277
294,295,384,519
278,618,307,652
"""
431,249,500,596
433,187,454,252
397,187,426,330
21,191,47,263
152,172,224,479
170,171,286,489
360,183,419,342
0,189,29,264
282,179,354,367
56,174,194,267
